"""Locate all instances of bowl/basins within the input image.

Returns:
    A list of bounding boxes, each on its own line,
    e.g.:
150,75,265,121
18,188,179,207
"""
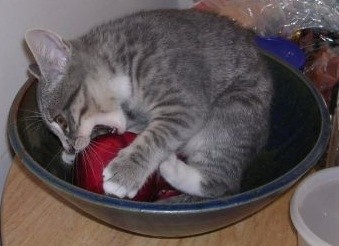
5,45,331,239
289,166,338,245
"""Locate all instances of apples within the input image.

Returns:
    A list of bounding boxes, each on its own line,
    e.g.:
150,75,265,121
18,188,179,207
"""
77,131,158,202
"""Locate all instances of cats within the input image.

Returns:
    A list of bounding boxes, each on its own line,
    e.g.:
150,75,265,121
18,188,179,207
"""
13,6,277,200
